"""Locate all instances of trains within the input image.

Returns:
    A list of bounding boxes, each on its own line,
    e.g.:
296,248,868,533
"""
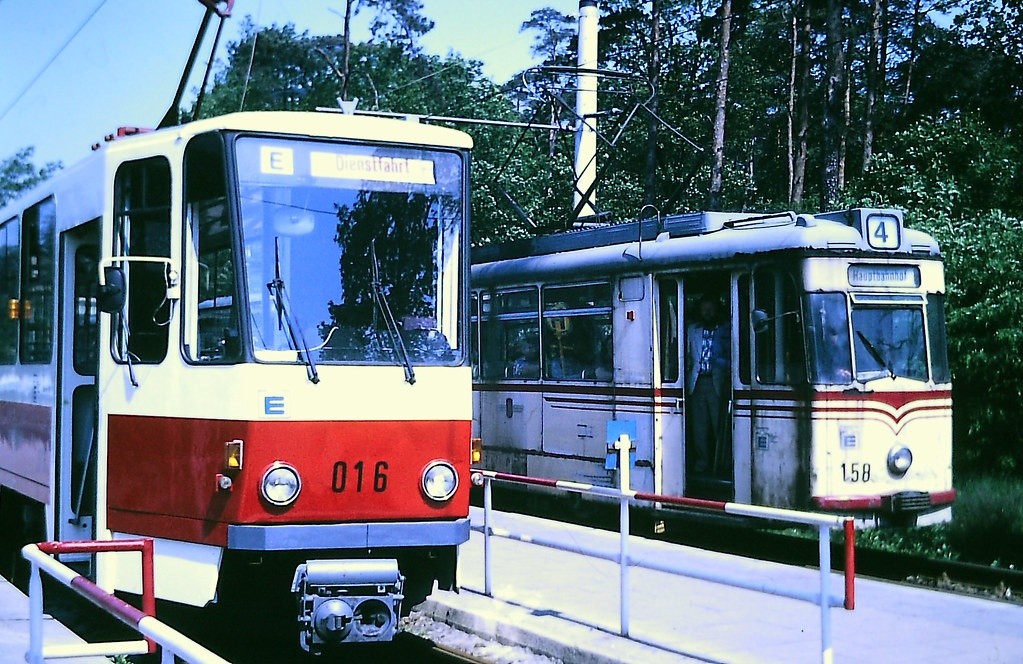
199,204,957,538
0,109,478,664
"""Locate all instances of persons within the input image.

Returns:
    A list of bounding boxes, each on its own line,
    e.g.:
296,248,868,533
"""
471,331,601,382
685,295,731,479
